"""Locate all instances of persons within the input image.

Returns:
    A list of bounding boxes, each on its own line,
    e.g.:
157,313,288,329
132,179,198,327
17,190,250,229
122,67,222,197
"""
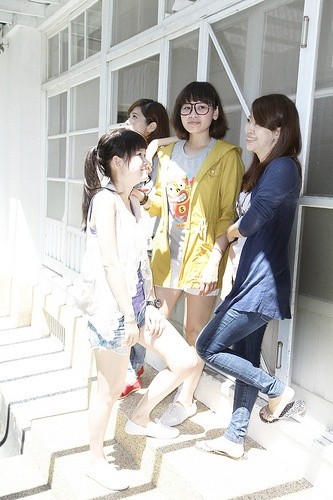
118,97,171,397
195,93,303,459
132,83,245,426
80,129,197,490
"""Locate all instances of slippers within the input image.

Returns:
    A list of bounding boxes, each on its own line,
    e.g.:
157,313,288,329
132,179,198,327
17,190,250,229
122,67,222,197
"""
259,400,305,423
196,435,244,459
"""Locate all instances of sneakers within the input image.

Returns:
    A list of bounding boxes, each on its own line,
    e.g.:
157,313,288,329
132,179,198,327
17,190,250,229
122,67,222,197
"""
125,418,180,439
86,460,129,490
160,399,198,427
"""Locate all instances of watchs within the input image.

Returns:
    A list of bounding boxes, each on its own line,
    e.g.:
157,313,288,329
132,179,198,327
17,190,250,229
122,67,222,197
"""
146,298,162,309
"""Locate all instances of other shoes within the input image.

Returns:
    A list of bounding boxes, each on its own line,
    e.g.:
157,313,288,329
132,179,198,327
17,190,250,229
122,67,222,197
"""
136,367,144,377
118,377,142,399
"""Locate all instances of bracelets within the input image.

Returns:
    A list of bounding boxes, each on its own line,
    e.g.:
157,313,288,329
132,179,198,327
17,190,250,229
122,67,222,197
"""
140,194,148,205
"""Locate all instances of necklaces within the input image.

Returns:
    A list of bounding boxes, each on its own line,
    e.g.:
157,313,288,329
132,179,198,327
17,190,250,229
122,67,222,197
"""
236,191,251,216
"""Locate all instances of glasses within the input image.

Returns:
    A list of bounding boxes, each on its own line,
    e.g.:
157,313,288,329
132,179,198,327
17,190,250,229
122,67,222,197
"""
179,101,214,116
